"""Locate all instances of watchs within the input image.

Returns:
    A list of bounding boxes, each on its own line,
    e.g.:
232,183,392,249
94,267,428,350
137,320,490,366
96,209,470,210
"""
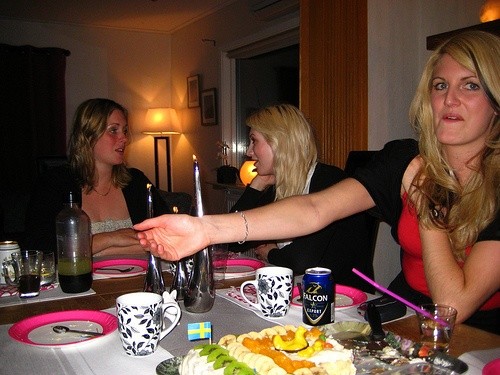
417,208,444,221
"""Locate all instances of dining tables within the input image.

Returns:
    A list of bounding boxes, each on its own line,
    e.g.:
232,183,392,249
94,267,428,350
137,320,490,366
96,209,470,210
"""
0,251,500,375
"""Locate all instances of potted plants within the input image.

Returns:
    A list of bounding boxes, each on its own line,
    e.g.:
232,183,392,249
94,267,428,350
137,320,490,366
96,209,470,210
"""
214,139,238,185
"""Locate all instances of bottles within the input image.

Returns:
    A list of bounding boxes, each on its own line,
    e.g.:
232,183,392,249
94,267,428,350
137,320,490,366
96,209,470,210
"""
55,192,93,293
0,241,22,284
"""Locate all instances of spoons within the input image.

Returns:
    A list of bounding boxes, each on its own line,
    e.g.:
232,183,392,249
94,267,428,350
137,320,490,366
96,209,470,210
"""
53,326,105,336
297,282,303,303
92,267,134,273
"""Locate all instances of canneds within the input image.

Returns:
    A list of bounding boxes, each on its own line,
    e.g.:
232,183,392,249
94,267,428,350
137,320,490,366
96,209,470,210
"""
301,266,335,326
0,241,21,284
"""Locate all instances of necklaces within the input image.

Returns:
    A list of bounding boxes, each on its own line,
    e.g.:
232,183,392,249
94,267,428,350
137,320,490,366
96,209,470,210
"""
95,185,111,197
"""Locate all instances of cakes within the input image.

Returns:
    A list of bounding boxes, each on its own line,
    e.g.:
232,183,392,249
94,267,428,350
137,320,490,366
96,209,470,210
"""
178,324,356,375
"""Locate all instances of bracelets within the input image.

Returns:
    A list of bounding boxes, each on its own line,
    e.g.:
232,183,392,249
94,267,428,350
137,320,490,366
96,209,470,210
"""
234,211,248,245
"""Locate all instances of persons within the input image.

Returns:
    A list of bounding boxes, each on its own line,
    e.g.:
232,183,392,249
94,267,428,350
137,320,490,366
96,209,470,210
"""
132,30,500,337
22,98,170,257
226,105,381,295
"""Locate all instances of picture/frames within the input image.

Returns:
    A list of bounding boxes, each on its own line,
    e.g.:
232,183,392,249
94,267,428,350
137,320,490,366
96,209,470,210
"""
187,74,200,109
200,88,218,126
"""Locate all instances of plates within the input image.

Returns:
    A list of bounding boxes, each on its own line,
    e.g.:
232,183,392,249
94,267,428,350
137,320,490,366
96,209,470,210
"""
212,258,265,280
291,283,367,311
7,309,119,348
92,258,149,276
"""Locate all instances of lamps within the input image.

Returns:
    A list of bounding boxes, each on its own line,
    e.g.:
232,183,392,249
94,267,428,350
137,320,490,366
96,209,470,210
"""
140,107,181,192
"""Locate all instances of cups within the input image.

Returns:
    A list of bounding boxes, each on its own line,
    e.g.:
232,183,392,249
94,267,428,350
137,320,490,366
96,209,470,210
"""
240,266,293,317
40,252,55,286
416,304,458,352
209,243,228,287
2,250,43,298
115,292,181,356
